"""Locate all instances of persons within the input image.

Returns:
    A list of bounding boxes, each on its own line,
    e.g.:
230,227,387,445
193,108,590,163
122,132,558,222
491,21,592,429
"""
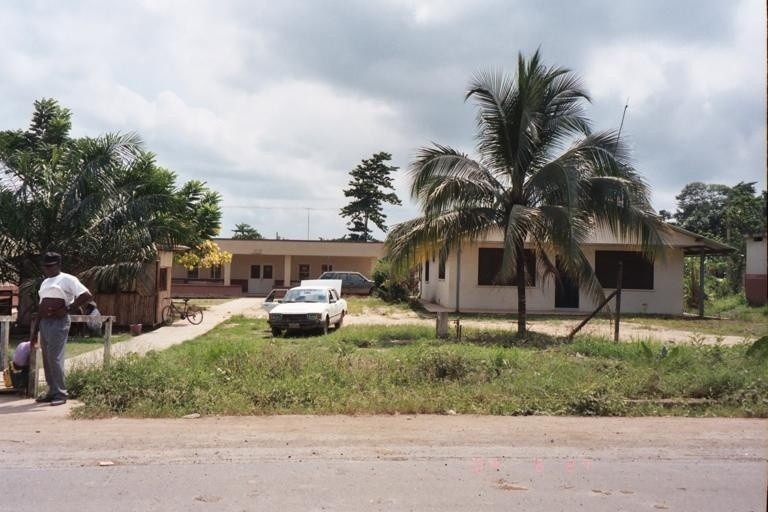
29,250,93,404
83,302,103,338
66,305,84,336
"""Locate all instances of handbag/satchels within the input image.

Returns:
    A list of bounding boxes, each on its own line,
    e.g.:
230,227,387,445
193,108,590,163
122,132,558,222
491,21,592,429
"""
39,297,65,318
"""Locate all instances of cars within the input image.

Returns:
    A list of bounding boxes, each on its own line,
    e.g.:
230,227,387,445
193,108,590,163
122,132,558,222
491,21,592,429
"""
261,278,348,337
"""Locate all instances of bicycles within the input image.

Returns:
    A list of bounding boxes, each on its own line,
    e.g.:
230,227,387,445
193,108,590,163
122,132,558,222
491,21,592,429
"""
162,295,204,326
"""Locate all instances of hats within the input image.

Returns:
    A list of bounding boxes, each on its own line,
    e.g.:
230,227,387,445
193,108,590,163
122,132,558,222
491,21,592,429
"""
41,251,60,267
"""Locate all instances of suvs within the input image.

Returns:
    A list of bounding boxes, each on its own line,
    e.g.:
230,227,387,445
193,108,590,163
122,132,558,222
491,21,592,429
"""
317,271,382,298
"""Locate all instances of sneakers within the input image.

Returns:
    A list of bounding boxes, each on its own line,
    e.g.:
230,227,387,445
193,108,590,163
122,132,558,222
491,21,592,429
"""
52,399,65,405
35,394,51,402
